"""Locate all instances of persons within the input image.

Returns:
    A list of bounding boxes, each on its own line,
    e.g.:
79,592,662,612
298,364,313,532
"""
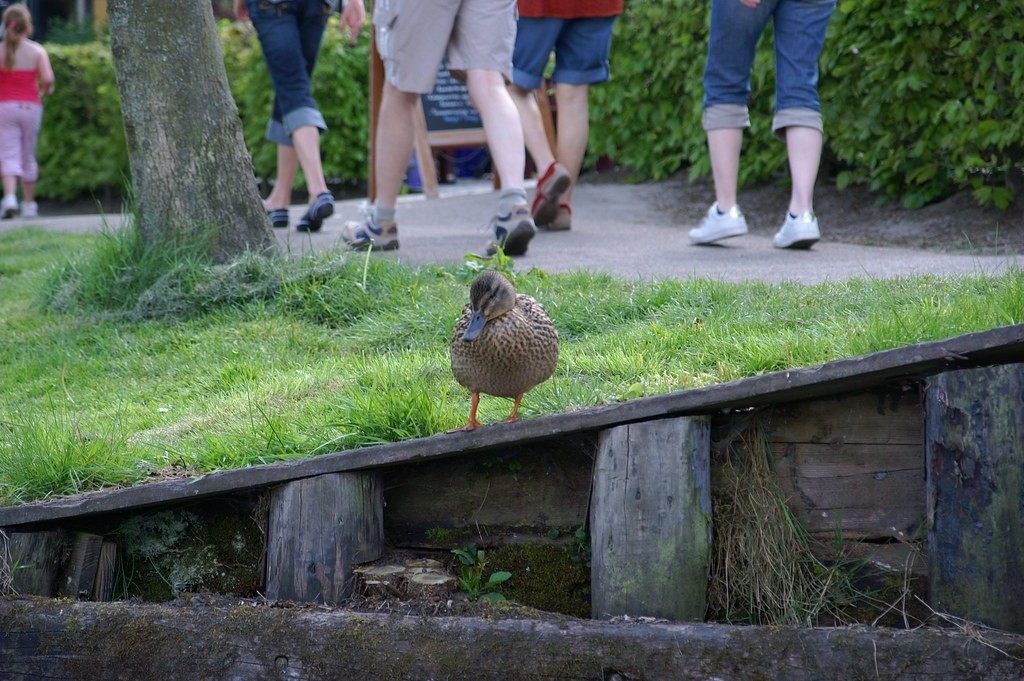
503,0,625,234
687,0,838,250
230,0,365,233
342,0,534,258
1,4,56,220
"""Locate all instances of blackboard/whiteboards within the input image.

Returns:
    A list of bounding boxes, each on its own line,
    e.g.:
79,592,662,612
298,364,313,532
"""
413,53,489,147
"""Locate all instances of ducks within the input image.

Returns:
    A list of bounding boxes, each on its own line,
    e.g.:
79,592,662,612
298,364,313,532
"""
446,271,560,432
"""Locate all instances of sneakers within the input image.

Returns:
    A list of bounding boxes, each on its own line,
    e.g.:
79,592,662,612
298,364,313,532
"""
688,201,749,244
297,192,334,232
531,161,572,230
773,211,820,248
342,215,399,250
0,195,38,218
486,204,534,256
267,209,289,228
